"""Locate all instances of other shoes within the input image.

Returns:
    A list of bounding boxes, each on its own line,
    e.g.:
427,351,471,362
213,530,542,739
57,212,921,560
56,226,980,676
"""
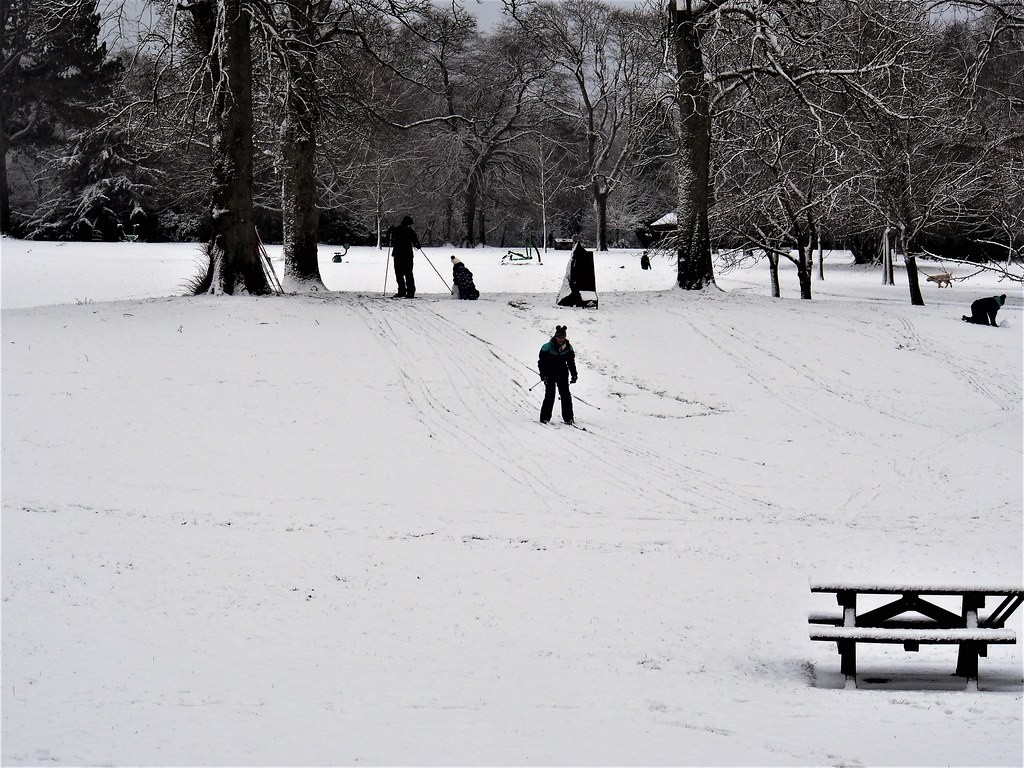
394,292,414,298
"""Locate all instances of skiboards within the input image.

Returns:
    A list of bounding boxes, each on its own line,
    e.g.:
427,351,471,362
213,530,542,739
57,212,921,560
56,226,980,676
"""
372,294,419,302
539,416,589,432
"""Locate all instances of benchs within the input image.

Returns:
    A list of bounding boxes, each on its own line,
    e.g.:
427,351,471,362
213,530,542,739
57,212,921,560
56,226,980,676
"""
807,607,1006,629
809,624,1018,646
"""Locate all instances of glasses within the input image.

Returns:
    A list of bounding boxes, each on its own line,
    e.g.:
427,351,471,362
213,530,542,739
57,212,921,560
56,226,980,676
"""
557,336,565,339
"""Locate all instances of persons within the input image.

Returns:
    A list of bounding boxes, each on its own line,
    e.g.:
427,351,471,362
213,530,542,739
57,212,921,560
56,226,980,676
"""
451,255,480,300
541,233,544,247
549,231,554,248
962,294,1006,328
538,325,578,426
641,251,652,270
385,216,421,298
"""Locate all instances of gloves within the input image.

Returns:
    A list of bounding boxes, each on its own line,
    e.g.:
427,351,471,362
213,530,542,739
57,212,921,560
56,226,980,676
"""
570,376,578,384
541,374,547,381
416,244,421,249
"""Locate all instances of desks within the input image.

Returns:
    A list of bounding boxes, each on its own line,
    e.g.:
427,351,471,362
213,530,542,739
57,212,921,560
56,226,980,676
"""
808,573,1023,691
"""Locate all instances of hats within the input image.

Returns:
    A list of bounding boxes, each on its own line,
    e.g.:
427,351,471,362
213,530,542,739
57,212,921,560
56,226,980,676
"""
401,216,413,225
555,326,567,337
451,256,461,265
999,294,1006,305
